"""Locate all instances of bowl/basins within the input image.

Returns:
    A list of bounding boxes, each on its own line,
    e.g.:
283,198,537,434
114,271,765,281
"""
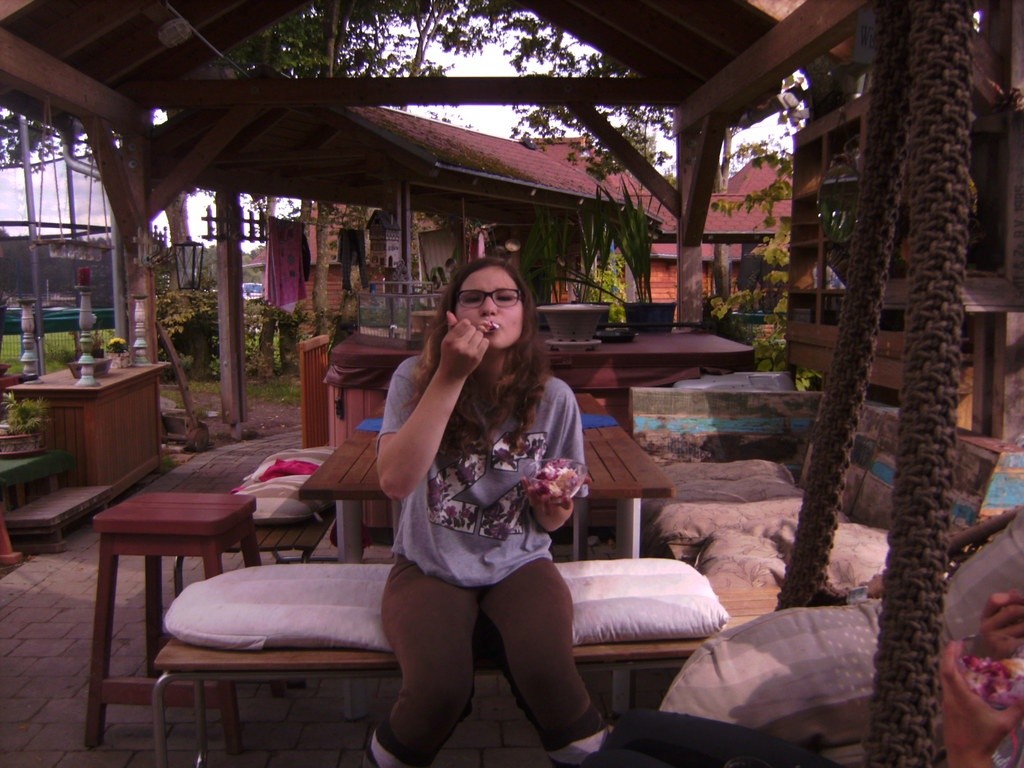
523,457,588,504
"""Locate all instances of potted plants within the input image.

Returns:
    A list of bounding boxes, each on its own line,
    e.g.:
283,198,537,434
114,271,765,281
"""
0,390,58,461
67,314,113,380
518,171,677,341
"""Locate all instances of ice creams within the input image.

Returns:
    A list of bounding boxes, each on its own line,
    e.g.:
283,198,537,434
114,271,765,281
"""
960,654,1024,708
534,459,582,501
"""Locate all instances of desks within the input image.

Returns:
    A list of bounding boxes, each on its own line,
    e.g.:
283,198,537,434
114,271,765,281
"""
294,392,675,563
732,309,782,331
9,360,174,509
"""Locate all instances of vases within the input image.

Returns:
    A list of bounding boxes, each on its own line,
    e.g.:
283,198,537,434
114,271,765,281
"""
103,351,134,370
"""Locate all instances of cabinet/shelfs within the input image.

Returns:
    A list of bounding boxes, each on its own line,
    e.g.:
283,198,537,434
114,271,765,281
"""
785,89,987,435
327,331,755,529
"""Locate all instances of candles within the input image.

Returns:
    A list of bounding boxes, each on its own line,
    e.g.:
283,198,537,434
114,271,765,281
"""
78,267,90,286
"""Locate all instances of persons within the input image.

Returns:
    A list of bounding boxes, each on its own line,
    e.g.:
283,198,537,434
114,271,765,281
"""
542,586,1024,767
354,254,612,768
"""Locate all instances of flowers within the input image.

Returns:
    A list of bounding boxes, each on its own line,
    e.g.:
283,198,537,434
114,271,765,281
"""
105,336,128,353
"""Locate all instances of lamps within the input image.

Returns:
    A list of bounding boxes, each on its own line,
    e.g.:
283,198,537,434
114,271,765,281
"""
144,225,205,294
816,136,863,245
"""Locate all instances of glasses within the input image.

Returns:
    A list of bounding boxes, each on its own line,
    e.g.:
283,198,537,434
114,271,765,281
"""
455,288,523,309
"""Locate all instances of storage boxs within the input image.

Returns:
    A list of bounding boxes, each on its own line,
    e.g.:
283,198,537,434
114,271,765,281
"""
882,111,1024,313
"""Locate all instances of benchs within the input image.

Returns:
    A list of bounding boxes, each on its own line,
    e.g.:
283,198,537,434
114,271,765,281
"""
5,485,113,552
150,557,730,768
620,385,1001,768
226,511,335,565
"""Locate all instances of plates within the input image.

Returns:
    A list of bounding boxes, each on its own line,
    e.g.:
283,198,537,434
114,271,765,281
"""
593,330,640,343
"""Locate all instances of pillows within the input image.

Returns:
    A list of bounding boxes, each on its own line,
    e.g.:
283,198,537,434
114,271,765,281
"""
659,601,880,752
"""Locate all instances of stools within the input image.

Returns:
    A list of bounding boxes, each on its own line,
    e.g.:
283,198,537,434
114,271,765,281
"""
81,490,263,758
0,448,74,503
